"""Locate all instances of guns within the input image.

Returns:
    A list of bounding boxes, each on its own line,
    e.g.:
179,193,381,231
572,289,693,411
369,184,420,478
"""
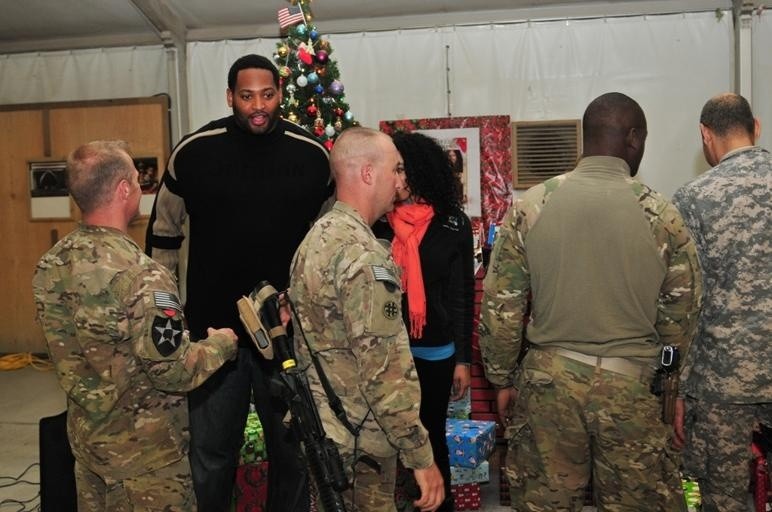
252,280,351,512
656,344,678,422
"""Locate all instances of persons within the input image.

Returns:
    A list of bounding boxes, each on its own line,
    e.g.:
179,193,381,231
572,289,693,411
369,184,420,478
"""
278,125,446,511
31,137,241,512
447,150,462,172
143,53,338,510
668,93,771,511
374,129,474,509
476,92,703,511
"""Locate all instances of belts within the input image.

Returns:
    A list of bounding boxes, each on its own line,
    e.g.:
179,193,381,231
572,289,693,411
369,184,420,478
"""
529,346,654,381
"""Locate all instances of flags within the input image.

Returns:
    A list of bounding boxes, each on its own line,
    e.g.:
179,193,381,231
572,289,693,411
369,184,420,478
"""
277,5,304,31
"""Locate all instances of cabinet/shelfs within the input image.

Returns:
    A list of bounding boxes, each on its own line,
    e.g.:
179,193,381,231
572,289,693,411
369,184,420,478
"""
0,93,172,360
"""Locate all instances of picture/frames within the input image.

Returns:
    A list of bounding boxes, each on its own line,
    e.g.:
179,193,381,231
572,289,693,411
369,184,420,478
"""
26,159,75,223
128,155,161,222
